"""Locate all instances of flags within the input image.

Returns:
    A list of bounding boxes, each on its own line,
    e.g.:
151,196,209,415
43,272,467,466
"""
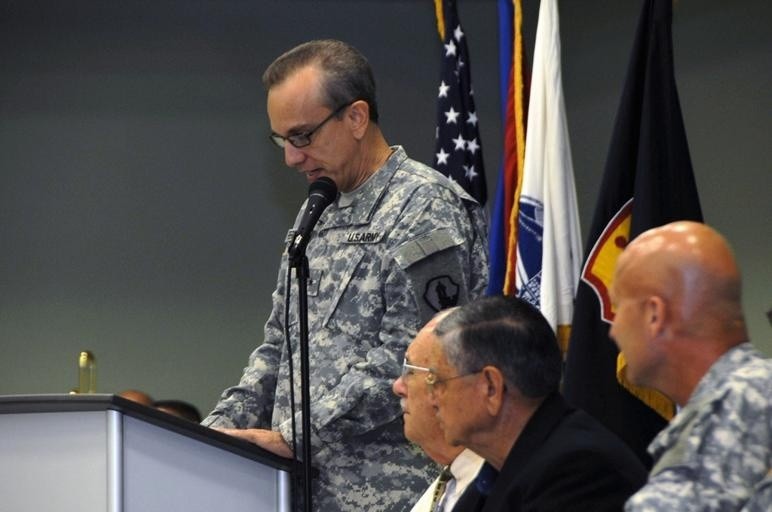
483,0,540,298
511,0,585,343
425,1,490,214
566,0,705,474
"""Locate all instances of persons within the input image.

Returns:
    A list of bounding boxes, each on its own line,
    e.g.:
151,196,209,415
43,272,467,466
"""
393,302,494,511
423,294,651,510
198,34,492,511
605,218,770,511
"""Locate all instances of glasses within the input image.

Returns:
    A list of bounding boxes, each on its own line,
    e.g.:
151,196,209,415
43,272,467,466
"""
400,359,436,379
424,370,482,395
268,98,361,150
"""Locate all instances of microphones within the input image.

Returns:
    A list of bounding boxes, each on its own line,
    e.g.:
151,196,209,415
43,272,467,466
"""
288,177,338,261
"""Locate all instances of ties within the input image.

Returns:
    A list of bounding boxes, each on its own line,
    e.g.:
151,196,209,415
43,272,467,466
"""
431,463,452,511
